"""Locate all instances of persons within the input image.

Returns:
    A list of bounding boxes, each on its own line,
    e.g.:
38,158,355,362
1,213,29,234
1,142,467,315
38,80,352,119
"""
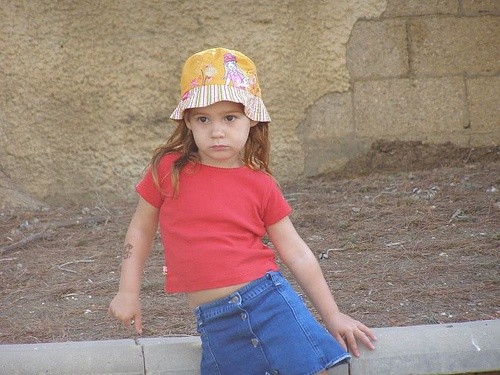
108,48,376,375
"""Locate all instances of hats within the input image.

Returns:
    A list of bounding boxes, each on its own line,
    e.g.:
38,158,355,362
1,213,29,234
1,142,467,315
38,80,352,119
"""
170,48,272,123
